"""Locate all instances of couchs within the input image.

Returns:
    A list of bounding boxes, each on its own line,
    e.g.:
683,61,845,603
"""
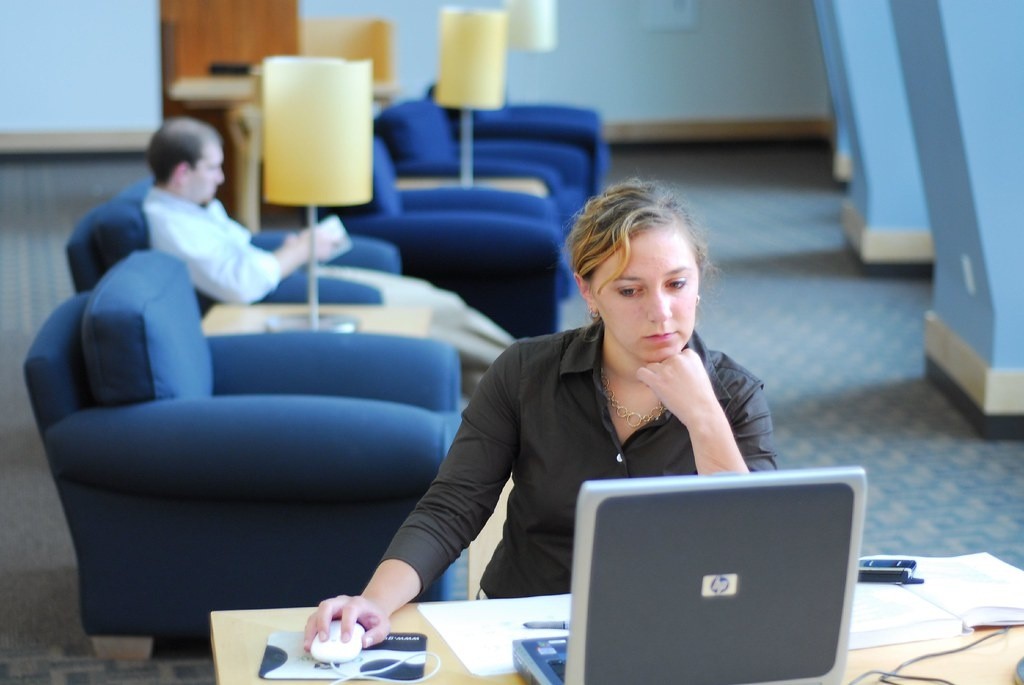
19,248,476,656
376,98,594,257
73,170,403,307
420,71,612,193
323,124,579,350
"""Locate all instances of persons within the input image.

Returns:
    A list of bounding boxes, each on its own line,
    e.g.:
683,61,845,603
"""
302,174,782,654
142,116,517,377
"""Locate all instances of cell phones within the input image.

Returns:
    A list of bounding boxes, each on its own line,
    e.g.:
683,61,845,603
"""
859,559,925,585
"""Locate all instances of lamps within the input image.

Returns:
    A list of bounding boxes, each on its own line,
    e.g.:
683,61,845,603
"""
508,2,561,107
257,53,382,337
436,6,514,189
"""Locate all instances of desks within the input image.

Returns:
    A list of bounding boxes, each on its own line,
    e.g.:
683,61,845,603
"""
208,544,1024,685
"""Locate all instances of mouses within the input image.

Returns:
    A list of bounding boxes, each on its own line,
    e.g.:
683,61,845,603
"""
310,620,366,664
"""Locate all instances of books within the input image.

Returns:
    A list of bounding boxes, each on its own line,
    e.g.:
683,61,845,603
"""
847,549,1023,650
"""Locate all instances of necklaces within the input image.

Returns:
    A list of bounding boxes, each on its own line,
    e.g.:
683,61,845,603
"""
601,364,669,428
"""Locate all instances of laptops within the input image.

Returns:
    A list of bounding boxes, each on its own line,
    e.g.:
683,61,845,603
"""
513,465,868,685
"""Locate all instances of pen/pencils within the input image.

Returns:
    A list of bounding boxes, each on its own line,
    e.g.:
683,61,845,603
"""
522,621,571,629
